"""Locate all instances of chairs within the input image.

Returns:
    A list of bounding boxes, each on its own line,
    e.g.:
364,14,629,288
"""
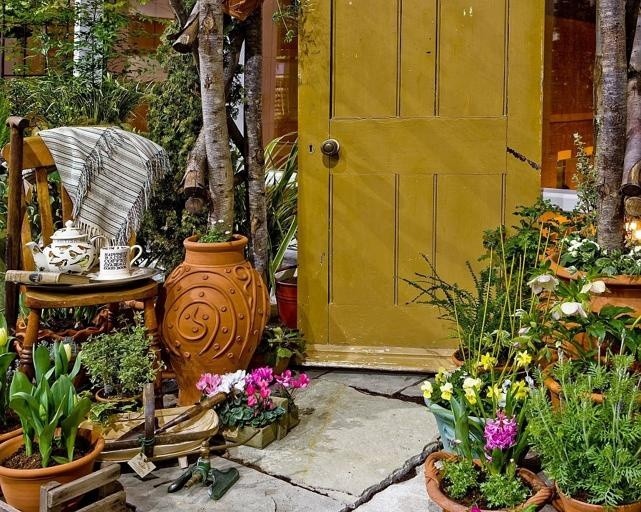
2,124,165,410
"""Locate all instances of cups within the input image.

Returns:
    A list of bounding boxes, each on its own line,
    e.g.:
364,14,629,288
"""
99,244,143,274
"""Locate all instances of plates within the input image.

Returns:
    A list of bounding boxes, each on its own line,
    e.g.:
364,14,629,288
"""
86,269,149,281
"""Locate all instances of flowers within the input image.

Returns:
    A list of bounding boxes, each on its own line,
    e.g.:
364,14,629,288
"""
195,365,309,427
196,218,233,243
424,237,640,512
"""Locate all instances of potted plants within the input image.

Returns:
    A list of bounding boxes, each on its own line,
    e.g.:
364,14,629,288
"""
0,312,167,512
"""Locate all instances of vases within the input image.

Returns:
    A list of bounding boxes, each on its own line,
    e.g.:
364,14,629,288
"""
219,423,279,449
159,236,271,410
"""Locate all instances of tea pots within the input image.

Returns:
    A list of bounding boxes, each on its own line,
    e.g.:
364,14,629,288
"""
25,219,109,274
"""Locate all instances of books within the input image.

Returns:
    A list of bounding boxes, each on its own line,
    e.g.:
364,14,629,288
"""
5,269,92,285
